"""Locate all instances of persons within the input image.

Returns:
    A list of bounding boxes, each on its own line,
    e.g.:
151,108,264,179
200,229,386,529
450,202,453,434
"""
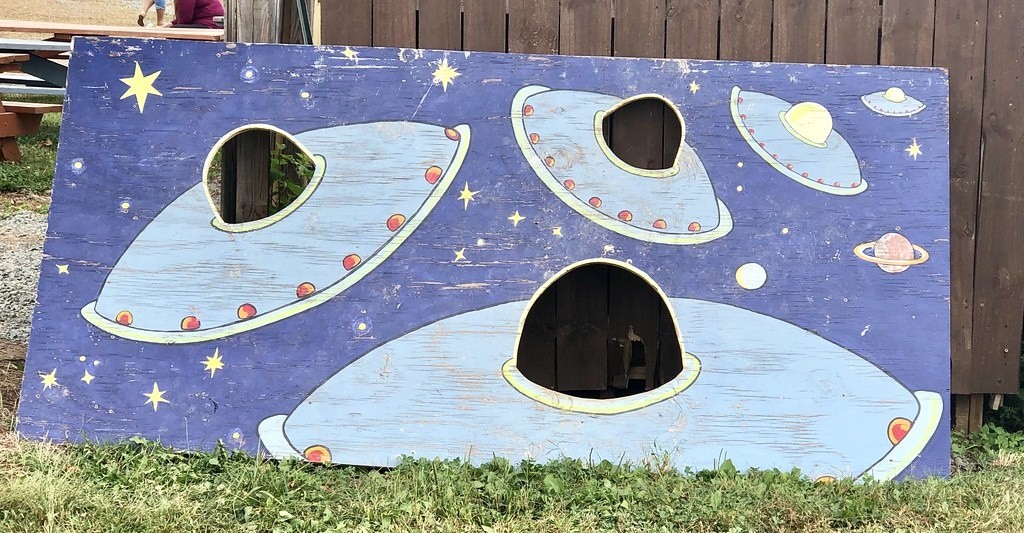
161,0,225,41
137,0,167,26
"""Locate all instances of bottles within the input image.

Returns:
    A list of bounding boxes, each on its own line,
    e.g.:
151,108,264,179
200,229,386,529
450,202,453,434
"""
146,20,155,39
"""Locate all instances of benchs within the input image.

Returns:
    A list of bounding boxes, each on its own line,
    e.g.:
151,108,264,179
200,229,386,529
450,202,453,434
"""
1,100,63,113
0,83,66,95
0,72,46,82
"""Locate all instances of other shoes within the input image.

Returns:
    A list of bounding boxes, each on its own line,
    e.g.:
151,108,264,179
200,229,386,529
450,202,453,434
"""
136,14,145,27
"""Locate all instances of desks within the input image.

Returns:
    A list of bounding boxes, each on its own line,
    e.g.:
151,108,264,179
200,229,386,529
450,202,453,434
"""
0,19,224,162
